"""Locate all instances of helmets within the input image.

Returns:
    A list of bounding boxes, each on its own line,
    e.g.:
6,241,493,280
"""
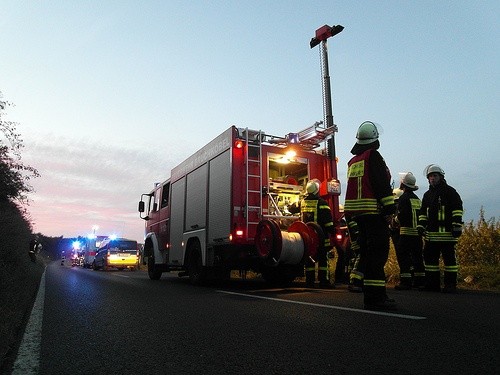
399,172,419,189
424,163,446,180
305,180,320,196
392,187,404,200
355,120,383,144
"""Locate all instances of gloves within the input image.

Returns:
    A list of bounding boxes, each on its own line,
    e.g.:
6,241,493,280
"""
417,227,424,236
452,224,462,238
393,219,399,228
328,225,337,236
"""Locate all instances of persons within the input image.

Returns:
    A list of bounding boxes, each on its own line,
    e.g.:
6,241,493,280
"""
61,251,65,265
29,235,39,263
344,121,398,310
389,188,403,267
301,178,336,289
392,172,426,290
347,216,364,292
417,165,463,292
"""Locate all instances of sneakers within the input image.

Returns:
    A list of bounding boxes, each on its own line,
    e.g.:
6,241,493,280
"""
348,283,363,292
394,284,410,290
363,297,398,311
306,282,331,289
417,285,458,293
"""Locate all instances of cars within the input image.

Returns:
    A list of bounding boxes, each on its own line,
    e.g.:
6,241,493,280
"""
92,239,140,272
79,237,106,268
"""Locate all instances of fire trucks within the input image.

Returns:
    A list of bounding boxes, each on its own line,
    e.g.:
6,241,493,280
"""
137,25,346,291
